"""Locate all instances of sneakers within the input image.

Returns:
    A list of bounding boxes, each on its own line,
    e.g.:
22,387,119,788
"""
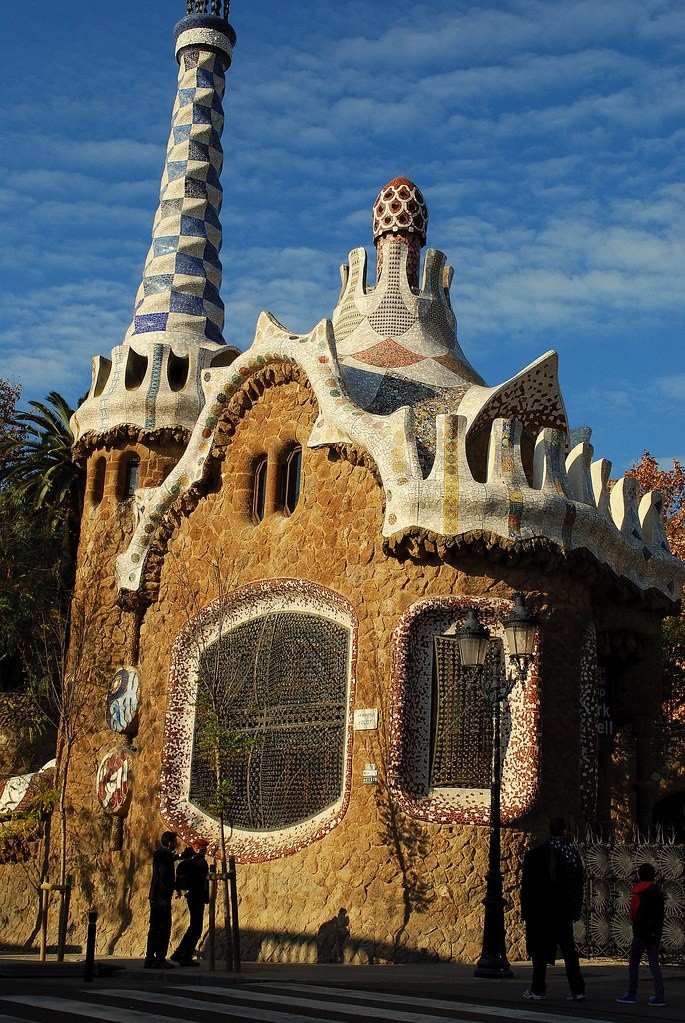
648,996,666,1006
521,990,544,1000
616,992,640,1003
564,992,587,1000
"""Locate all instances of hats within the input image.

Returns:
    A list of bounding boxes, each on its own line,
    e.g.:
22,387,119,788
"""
192,839,209,853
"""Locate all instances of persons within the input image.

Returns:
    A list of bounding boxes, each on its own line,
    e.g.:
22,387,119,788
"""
144,831,188,969
616,863,668,1007
519,815,588,1001
170,839,210,967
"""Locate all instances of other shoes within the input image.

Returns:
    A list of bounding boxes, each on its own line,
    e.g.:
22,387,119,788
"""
170,951,200,967
144,956,175,969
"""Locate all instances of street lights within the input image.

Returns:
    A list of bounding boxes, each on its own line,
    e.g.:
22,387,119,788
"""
452,591,538,978
